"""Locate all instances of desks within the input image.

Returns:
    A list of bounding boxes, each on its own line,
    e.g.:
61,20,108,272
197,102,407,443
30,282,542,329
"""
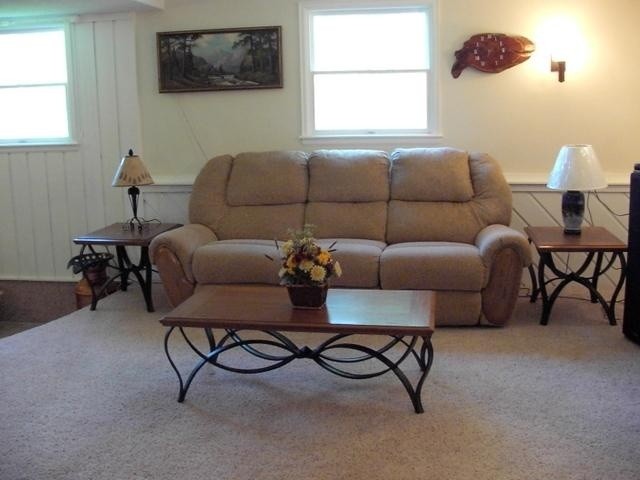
522,226,629,326
72,223,182,313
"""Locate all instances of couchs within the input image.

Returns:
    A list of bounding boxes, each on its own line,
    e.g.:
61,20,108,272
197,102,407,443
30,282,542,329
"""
147,147,530,328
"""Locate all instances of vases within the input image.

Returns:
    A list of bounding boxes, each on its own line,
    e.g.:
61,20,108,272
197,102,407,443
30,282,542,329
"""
288,280,329,310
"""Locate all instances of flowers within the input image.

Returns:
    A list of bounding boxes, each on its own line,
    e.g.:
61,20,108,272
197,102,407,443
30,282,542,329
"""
268,224,342,289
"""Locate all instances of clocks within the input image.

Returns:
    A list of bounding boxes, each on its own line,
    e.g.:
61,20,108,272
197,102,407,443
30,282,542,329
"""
451,34,535,79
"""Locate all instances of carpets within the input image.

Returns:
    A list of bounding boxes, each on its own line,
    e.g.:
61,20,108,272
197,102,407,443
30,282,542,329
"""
2,283,640,480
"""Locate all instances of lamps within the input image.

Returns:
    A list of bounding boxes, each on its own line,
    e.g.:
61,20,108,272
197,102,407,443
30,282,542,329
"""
111,148,160,232
547,40,570,82
547,144,608,235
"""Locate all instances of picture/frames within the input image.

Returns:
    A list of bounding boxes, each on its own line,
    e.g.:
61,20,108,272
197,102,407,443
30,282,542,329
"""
155,25,283,94
